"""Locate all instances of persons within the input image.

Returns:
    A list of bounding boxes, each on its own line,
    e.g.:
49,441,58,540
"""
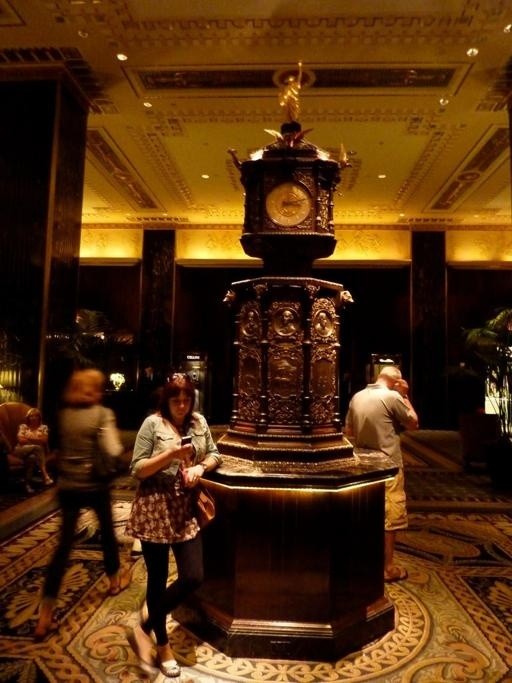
13,407,53,494
344,364,418,582
124,373,223,682
34,369,130,640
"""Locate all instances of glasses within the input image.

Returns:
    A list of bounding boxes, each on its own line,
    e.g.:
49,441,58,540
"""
167,372,192,383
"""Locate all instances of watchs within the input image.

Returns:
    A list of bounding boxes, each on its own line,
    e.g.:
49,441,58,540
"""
199,462,207,473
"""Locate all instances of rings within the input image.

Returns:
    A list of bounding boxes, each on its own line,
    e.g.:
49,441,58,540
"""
193,476,196,480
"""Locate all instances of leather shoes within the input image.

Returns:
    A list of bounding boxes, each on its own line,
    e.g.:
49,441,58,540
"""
126,626,159,676
161,658,181,677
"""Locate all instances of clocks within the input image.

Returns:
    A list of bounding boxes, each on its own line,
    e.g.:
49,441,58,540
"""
214,63,361,469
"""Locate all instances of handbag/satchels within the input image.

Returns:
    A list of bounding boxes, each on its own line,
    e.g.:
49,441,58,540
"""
191,484,216,529
93,440,129,482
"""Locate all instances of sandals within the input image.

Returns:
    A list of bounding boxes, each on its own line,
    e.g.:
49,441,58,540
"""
33,620,63,640
384,566,408,582
110,575,130,595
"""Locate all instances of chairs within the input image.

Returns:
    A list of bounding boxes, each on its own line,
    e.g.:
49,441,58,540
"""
1,400,57,492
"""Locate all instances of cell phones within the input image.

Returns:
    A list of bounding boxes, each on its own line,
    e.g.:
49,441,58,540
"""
181,436,192,446
